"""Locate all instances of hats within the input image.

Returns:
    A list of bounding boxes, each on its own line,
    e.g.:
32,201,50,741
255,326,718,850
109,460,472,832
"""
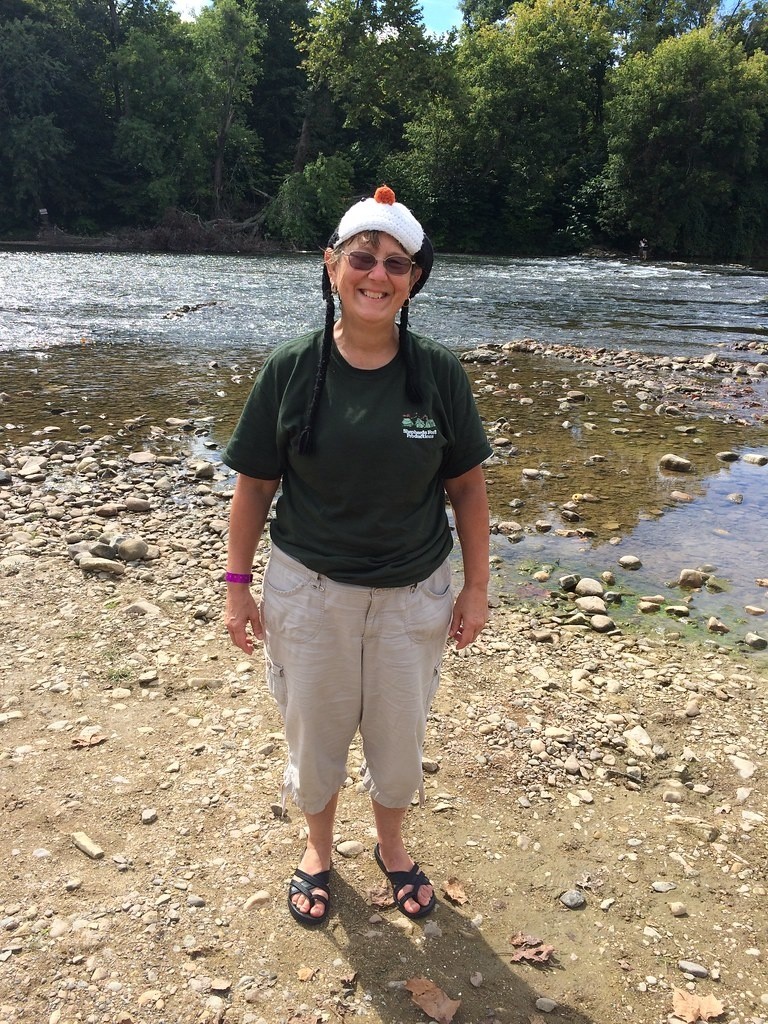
296,185,434,448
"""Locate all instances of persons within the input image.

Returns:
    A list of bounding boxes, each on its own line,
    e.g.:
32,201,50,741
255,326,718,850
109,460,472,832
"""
220,185,492,927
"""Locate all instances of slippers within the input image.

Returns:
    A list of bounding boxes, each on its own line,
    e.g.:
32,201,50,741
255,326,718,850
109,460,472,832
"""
288,846,331,923
374,843,436,918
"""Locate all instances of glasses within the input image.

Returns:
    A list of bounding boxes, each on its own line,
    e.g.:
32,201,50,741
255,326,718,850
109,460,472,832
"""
340,249,416,276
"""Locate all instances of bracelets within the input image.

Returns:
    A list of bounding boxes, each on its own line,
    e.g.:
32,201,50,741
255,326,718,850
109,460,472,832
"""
225,572,253,584
